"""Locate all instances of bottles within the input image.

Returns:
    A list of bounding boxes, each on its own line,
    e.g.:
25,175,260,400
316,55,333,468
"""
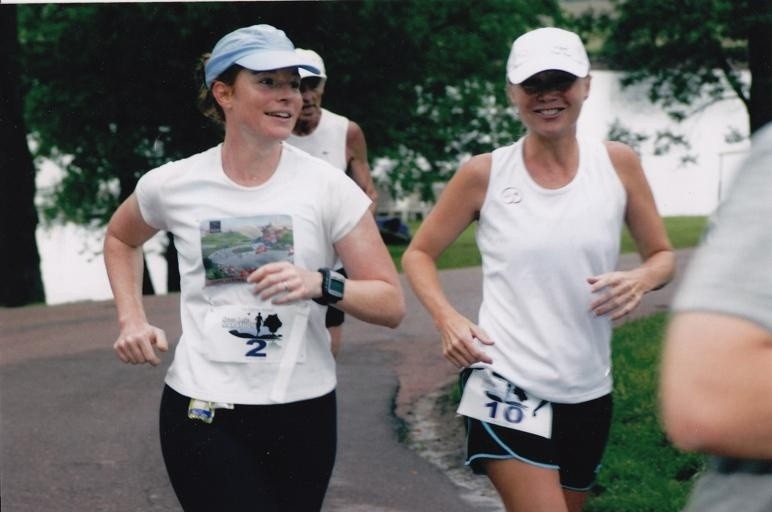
205,24,328,90
506,27,590,84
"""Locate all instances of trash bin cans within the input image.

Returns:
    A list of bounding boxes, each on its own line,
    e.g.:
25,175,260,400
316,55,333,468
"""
312,267,345,305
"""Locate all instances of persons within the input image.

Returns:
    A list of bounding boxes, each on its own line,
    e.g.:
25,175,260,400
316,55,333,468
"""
286,47,378,359
661,120,772,512
103,24,406,511
401,27,677,512
